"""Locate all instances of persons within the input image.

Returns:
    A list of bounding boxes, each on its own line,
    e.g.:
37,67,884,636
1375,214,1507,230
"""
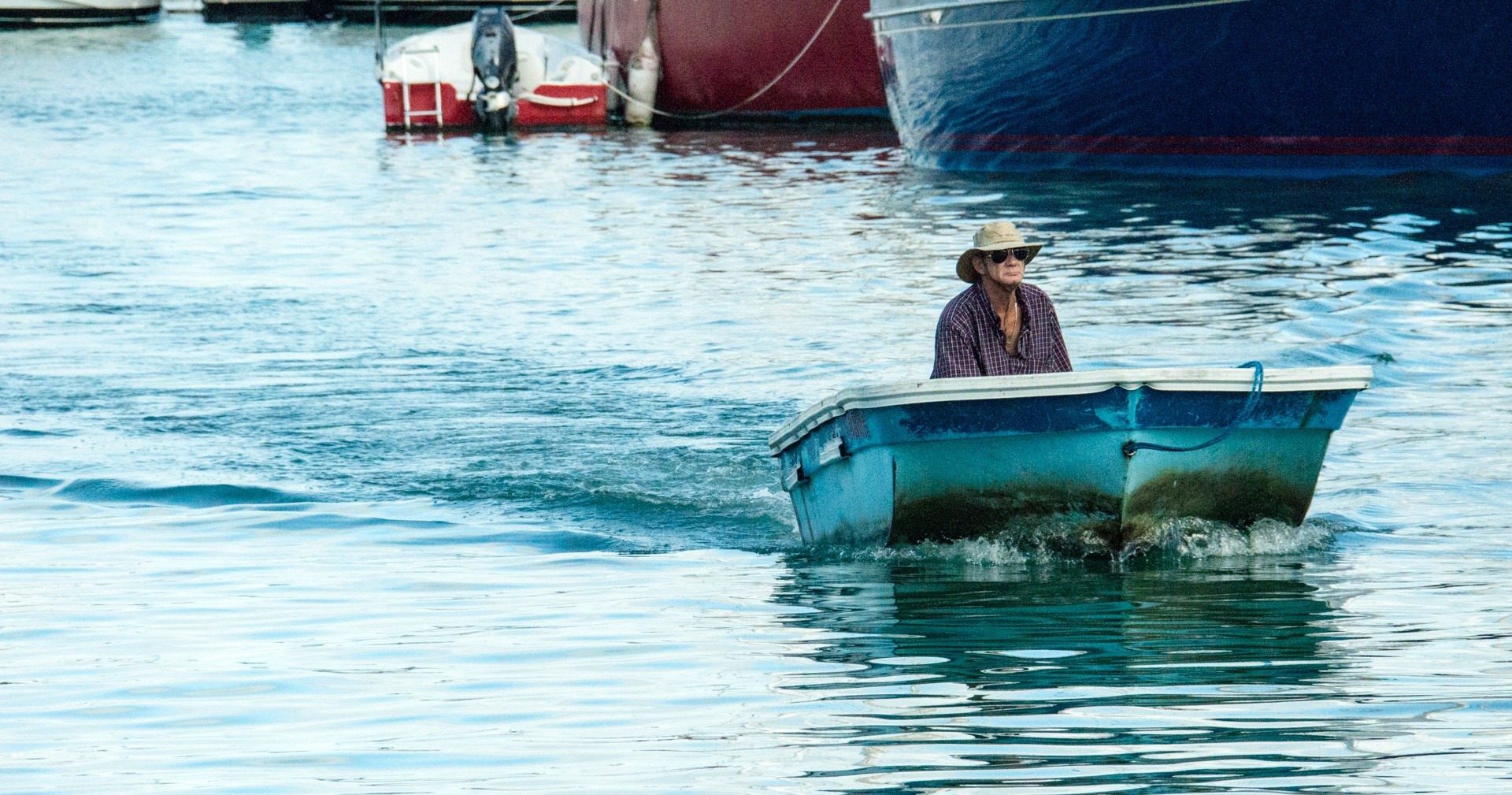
930,221,1073,379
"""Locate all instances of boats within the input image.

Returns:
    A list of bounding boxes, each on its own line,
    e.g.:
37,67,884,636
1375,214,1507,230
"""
766,365,1373,549
575,1,892,125
869,0,1512,184
378,19,609,134
200,0,580,24
0,0,162,29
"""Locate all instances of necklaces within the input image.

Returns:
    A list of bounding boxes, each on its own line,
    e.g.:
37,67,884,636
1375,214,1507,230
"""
1006,302,1017,345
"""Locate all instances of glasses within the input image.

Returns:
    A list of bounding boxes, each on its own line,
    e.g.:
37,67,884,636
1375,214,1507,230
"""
984,249,1030,264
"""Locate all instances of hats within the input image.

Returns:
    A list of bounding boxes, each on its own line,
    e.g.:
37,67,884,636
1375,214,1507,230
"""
956,220,1042,283
